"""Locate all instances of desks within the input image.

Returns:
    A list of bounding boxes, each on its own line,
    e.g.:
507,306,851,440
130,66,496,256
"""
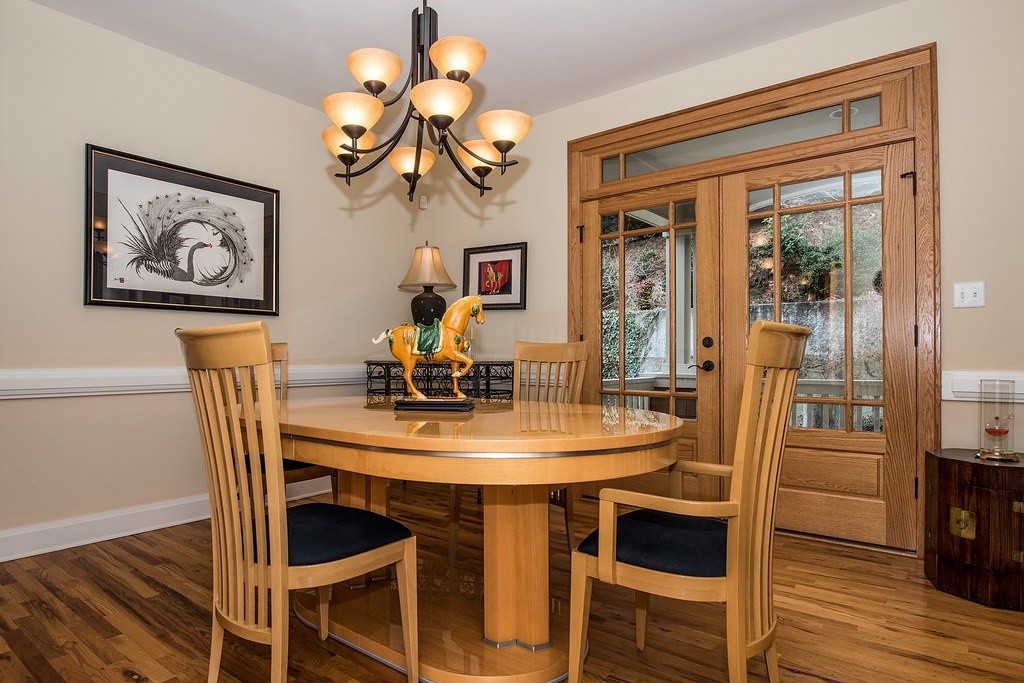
220,396,684,683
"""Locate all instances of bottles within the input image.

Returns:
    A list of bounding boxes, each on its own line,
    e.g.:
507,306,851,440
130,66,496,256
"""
466,326,478,361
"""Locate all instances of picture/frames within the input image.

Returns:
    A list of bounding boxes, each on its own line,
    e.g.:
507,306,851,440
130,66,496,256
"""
84,142,280,316
463,242,527,310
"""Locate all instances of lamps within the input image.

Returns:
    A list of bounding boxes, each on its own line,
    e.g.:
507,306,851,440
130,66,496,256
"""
974,379,1022,462
321,0,532,202
398,240,458,326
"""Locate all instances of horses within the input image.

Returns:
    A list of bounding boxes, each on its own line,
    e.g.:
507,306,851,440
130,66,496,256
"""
371,295,486,401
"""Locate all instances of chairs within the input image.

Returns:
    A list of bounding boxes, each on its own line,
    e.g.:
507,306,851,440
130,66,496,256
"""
175,320,418,683
568,319,813,683
209,343,337,503
448,340,591,566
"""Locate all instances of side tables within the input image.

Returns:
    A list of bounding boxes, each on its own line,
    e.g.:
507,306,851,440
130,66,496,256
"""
924,448,1024,612
